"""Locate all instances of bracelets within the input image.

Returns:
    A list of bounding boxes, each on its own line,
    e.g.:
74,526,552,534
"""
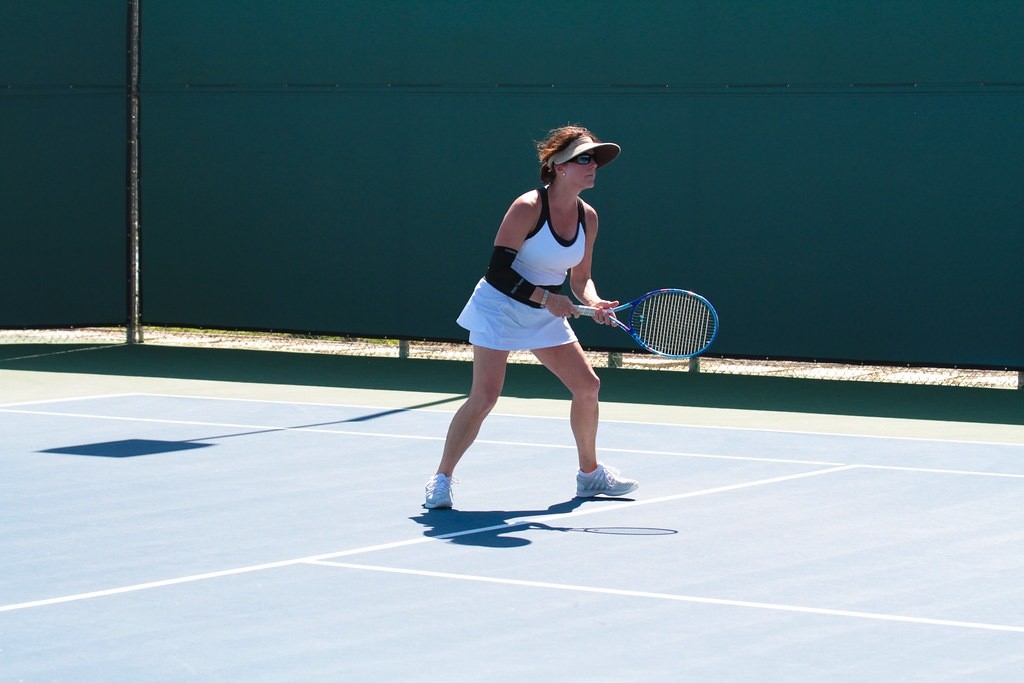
540,290,549,309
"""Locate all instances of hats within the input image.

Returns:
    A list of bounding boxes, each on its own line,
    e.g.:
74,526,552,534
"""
547,136,621,172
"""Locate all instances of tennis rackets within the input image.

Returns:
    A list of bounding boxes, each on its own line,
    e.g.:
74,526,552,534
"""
570,288,720,360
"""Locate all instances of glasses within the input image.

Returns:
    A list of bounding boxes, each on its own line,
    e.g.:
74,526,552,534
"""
566,152,597,164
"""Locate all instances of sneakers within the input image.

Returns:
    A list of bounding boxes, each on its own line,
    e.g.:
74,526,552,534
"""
576,464,639,497
425,473,459,508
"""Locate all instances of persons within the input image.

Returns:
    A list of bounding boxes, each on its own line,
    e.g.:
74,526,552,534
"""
425,125,639,509
